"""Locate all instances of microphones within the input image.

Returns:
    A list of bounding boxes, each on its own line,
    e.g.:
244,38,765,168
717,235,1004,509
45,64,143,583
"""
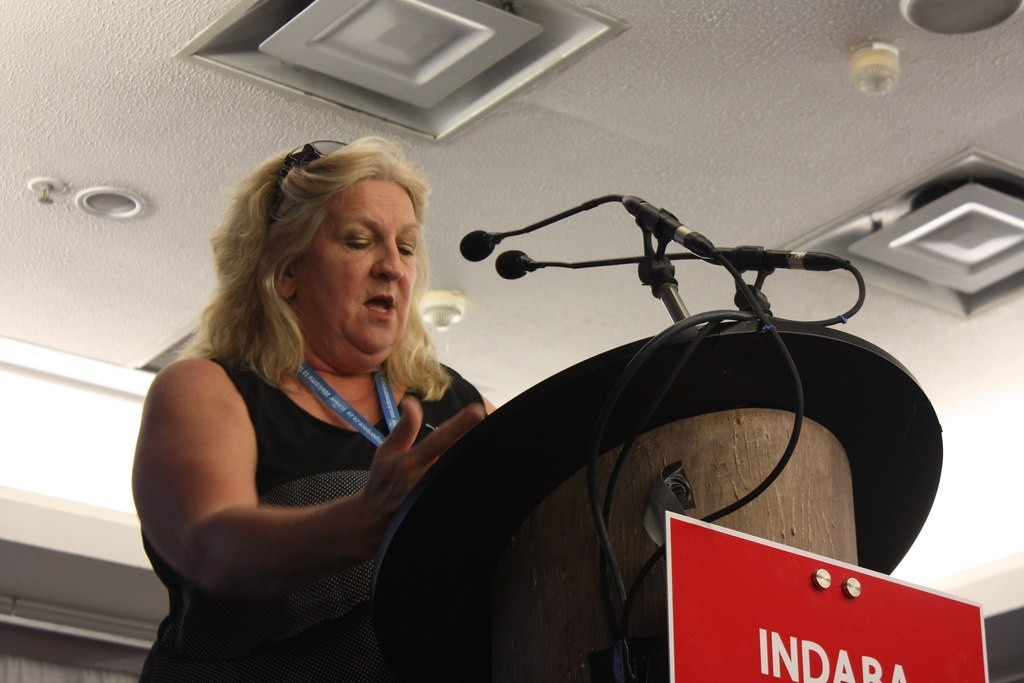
459,193,865,331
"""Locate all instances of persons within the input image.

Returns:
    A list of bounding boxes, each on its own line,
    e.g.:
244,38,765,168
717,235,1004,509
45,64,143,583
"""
114,129,496,683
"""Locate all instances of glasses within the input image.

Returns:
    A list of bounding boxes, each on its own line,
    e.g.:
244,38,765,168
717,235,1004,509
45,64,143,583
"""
293,140,348,166
